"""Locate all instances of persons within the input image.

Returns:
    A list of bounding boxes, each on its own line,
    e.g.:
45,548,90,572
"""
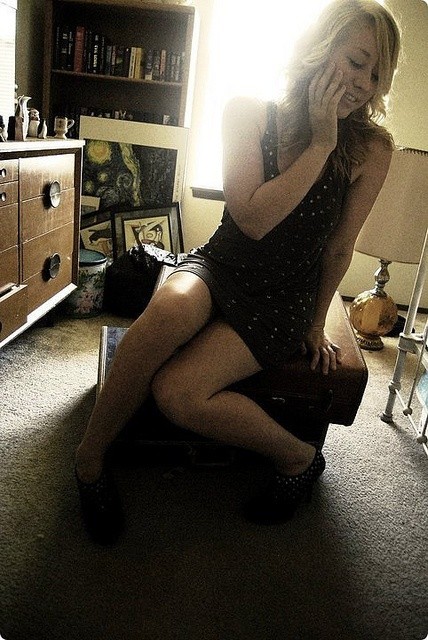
74,0,401,548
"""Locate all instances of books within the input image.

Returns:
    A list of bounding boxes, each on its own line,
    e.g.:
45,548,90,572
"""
52,23,185,82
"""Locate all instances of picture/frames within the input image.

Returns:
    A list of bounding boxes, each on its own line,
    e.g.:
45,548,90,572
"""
111,202,185,260
77,207,134,259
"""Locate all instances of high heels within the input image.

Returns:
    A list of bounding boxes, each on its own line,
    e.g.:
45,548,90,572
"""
238,450,325,524
74,459,123,550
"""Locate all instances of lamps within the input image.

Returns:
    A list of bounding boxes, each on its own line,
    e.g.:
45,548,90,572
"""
348,143,428,352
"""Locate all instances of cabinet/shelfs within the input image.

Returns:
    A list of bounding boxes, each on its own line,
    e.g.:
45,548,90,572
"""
42,2,196,201
20,158,87,350
0,158,28,342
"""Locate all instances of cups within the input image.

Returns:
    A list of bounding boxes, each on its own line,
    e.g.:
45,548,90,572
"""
55,117,74,139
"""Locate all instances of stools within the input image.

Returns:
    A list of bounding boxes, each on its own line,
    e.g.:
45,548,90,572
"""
153,263,368,467
